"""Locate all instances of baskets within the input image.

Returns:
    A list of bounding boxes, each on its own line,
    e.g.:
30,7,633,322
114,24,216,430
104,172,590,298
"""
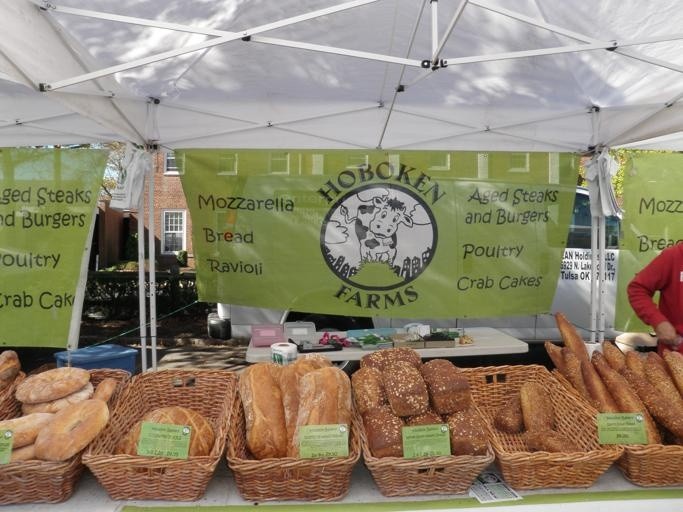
456,366,624,488
0,369,130,505
356,373,493,497
81,370,235,501
549,368,683,487
228,364,359,500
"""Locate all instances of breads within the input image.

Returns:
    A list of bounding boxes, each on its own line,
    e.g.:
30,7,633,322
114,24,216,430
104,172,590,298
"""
538,312,683,448
119,406,214,475
351,347,490,456
0,312,119,463
238,355,352,459
495,381,579,453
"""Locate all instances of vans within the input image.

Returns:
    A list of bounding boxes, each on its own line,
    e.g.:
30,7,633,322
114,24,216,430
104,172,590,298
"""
205,171,658,374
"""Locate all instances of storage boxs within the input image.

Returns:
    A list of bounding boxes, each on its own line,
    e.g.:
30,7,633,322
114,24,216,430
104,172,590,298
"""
54,342,137,374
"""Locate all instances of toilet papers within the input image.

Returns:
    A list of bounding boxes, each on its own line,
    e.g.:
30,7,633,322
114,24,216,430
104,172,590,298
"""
271,342,297,364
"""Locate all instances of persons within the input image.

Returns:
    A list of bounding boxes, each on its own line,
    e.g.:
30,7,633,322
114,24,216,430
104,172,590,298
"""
626,241,682,360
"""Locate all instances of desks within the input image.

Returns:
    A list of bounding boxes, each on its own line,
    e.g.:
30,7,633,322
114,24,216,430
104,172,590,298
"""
0,459,683,511
247,327,529,363
